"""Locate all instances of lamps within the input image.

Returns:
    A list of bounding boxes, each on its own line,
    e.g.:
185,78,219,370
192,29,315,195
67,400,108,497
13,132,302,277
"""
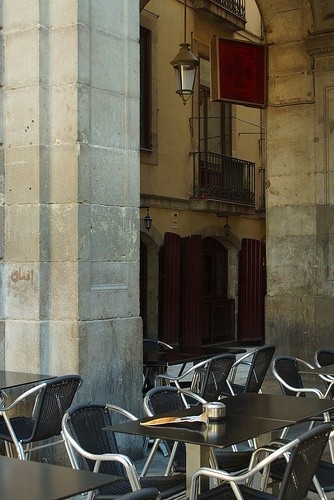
170,0,200,106
217,214,231,237
140,205,152,231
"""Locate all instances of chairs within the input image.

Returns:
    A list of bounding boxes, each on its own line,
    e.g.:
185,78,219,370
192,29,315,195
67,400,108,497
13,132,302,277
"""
0,341,334,500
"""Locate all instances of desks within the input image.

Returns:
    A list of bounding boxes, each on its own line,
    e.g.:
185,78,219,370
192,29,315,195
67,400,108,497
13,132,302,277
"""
196,392,334,495
0,455,123,500
102,408,295,500
0,371,57,390
297,363,334,375
143,349,246,388
